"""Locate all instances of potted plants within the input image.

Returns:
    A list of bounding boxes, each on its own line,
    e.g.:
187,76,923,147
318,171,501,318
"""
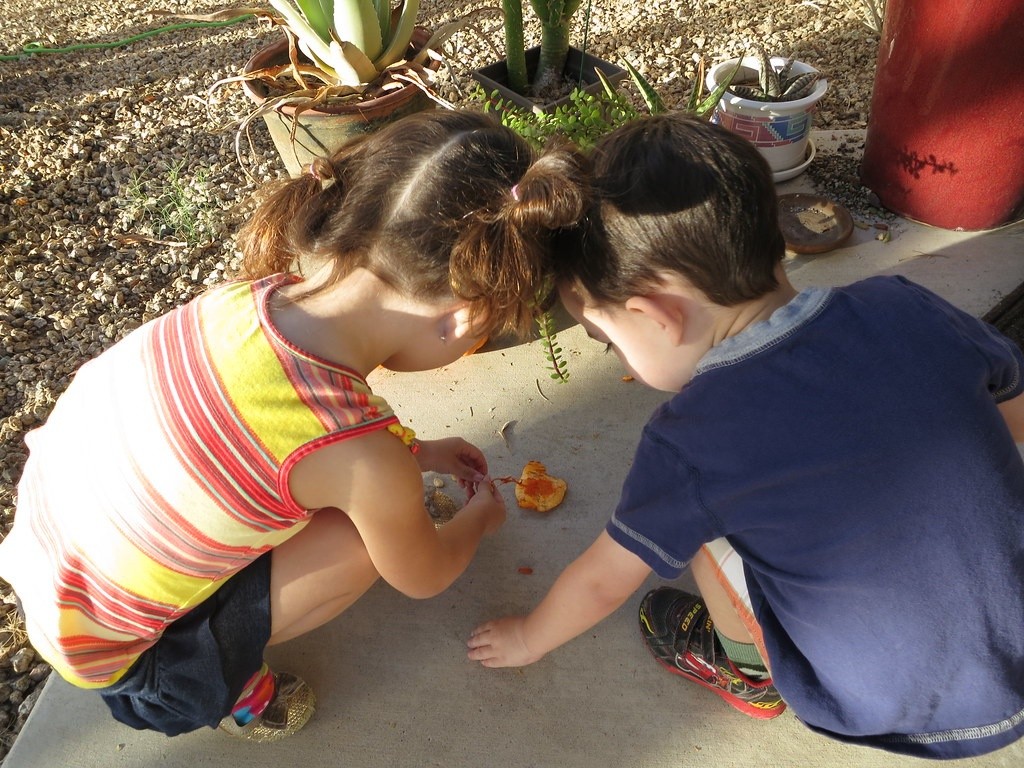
471,0,627,121
705,46,834,180
241,0,442,181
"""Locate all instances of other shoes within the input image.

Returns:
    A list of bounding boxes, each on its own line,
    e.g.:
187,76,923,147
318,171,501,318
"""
218,660,315,744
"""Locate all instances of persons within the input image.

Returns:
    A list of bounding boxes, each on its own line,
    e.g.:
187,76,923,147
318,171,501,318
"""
0,109,593,744
468,105,1024,757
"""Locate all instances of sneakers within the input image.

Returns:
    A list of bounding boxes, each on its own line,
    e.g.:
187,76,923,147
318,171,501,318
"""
638,586,786,720
